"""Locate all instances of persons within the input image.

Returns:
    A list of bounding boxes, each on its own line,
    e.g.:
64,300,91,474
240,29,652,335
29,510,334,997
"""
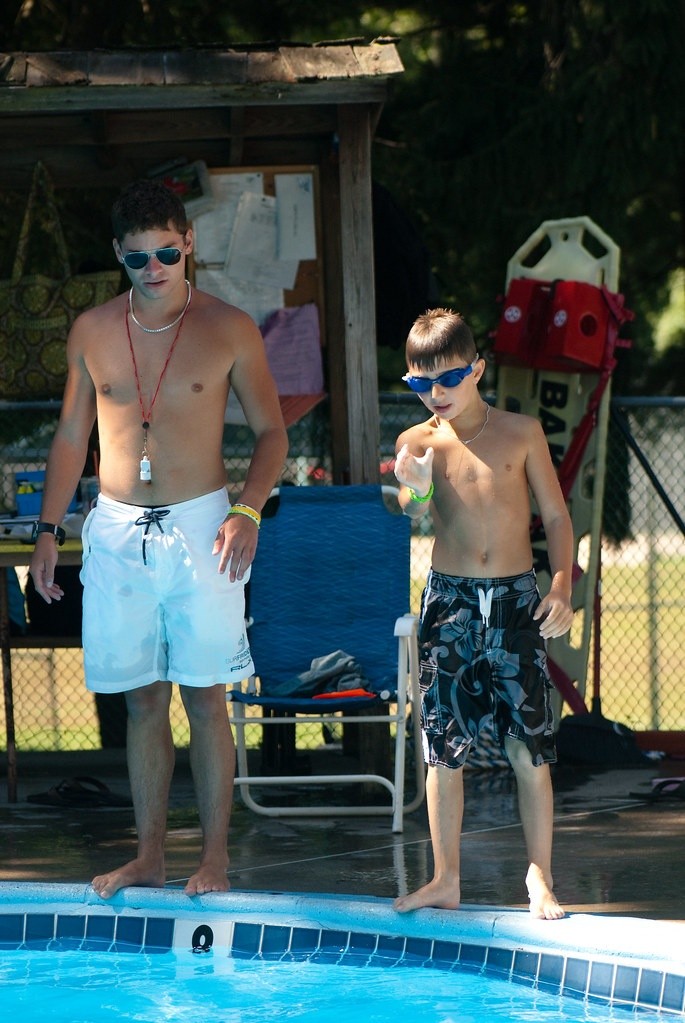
30,182,288,899
394,311,574,918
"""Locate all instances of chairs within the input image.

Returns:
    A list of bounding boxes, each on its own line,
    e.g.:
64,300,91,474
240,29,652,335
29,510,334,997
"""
225,484,425,833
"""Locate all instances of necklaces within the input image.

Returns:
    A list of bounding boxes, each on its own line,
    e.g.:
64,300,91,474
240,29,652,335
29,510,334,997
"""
434,402,490,444
125,292,187,480
128,277,192,333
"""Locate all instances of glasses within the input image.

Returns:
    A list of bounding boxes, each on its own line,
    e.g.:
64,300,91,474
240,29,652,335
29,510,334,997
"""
119,244,185,270
401,353,479,393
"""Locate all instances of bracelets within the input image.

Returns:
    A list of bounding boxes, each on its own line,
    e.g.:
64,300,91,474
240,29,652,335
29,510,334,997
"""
33,522,65,546
229,504,261,526
410,482,435,501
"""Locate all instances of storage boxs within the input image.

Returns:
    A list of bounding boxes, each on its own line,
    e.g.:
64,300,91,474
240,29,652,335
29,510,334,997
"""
14,469,77,515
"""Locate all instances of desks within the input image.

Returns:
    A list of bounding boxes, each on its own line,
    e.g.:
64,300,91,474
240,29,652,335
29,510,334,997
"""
0,536,85,566
